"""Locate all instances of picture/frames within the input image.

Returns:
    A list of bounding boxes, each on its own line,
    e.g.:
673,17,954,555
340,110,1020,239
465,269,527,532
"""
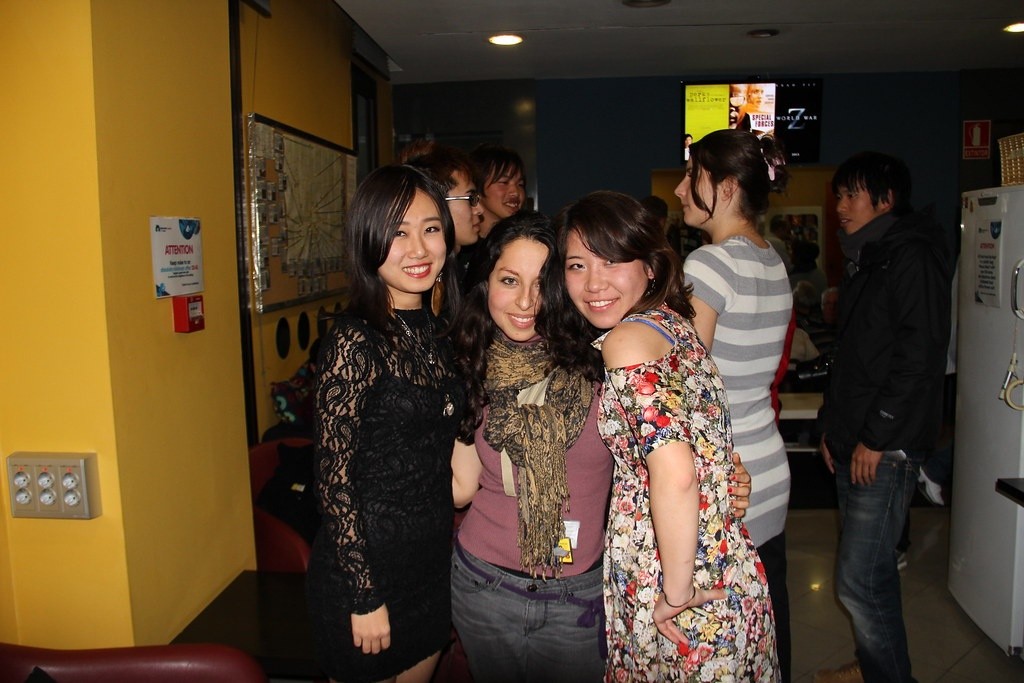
247,112,360,315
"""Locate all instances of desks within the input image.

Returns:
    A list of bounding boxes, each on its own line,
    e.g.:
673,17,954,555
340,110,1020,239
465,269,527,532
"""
165,569,332,683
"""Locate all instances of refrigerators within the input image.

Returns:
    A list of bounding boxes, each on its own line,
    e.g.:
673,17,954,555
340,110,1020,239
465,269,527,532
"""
948,186,1024,653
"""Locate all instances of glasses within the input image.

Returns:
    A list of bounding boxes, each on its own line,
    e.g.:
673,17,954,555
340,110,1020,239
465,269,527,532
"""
446,195,480,207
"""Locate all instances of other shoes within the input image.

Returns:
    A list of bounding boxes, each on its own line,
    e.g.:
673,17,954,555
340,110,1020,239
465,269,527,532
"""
895,550,908,568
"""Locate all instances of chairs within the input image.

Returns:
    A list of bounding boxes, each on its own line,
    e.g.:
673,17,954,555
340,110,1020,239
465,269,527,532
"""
249,437,326,573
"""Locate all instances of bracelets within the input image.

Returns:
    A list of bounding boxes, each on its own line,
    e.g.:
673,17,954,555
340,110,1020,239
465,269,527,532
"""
665,586,696,607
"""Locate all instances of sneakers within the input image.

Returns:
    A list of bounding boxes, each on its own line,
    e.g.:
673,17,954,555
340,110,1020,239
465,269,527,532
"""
811,661,864,683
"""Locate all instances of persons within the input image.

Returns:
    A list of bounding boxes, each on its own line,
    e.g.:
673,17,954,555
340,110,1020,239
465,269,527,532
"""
546,191,783,683
432,215,751,683
637,129,796,683
641,196,827,354
408,145,526,289
816,152,955,682
306,167,490,683
685,84,772,161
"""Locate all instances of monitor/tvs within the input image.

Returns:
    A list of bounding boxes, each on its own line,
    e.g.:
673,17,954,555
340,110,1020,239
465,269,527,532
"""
679,76,823,165
